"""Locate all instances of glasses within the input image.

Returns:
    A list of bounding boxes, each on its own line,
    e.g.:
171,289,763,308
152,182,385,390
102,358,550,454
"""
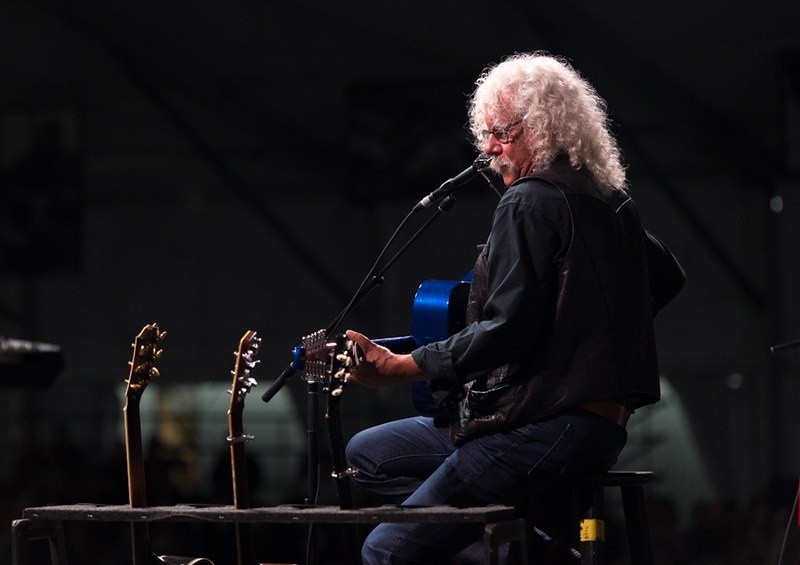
482,118,525,144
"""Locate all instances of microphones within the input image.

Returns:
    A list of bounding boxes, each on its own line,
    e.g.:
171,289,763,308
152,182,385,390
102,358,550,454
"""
413,153,492,212
438,166,497,212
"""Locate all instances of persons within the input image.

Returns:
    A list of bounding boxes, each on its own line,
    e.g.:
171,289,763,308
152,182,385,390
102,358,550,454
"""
340,51,685,565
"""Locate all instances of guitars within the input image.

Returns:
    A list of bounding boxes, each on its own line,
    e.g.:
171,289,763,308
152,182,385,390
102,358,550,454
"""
292,267,477,418
226,329,263,565
306,329,360,565
122,323,167,564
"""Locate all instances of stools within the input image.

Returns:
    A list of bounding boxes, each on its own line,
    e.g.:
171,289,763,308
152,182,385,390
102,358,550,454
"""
575,471,655,565
